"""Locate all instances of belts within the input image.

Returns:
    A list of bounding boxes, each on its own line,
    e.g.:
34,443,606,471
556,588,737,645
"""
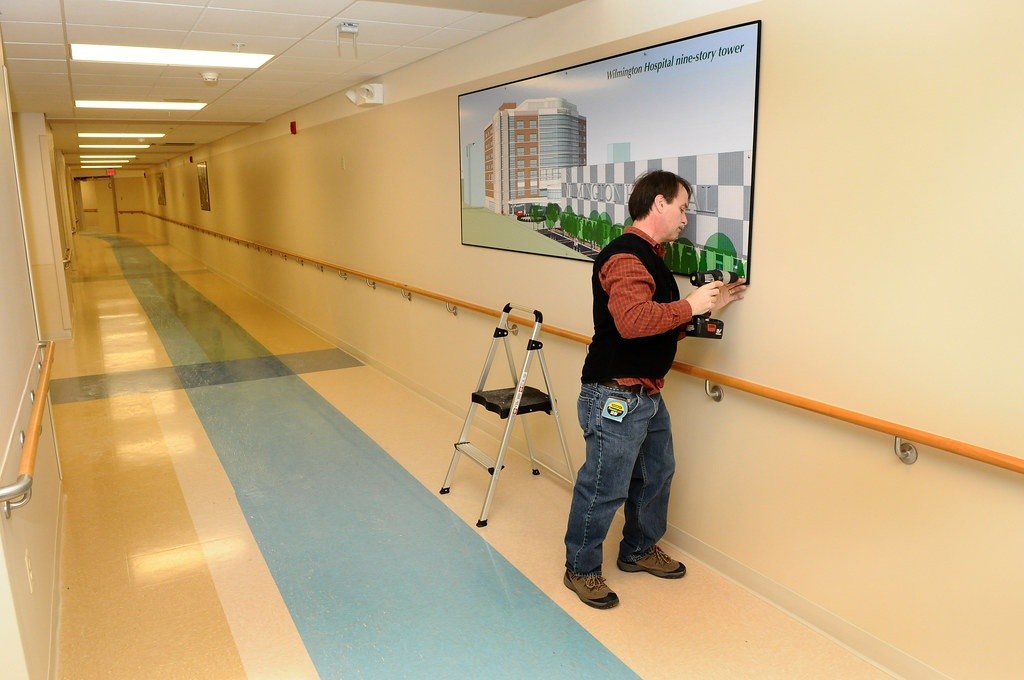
597,379,649,395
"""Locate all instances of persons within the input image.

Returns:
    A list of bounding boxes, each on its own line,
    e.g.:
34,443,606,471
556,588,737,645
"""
563,169,748,611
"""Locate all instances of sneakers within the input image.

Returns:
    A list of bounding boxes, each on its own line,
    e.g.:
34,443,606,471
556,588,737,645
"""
617,545,686,578
564,569,620,609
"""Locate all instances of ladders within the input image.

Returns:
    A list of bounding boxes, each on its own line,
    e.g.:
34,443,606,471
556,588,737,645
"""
439,302,579,527
